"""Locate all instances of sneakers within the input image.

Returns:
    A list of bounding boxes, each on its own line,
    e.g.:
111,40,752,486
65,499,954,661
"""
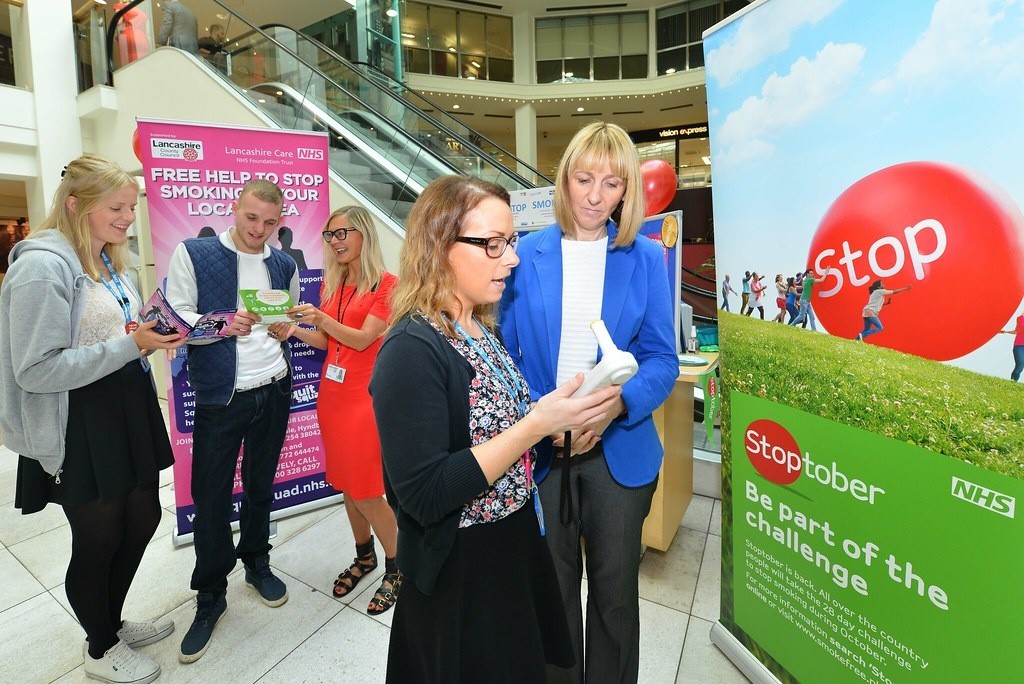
178,597,227,664
244,564,289,607
83,620,174,656
85,640,161,684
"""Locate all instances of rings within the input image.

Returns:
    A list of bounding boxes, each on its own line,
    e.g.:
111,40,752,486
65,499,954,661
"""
266,330,277,340
294,314,302,318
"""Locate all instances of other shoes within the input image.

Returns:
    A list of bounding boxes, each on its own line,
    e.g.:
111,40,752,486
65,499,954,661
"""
859,333,862,341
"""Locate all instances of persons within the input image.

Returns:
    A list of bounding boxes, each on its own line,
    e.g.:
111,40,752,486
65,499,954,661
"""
165,180,299,664
721,274,738,312
999,314,1024,382
0,153,188,684
337,370,342,380
158,0,198,56
856,279,911,343
366,176,624,684
198,24,225,76
113,0,151,70
493,122,680,684
268,205,402,616
740,271,767,320
771,266,831,331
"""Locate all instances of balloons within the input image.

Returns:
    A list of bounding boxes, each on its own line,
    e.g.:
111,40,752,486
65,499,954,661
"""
639,160,677,218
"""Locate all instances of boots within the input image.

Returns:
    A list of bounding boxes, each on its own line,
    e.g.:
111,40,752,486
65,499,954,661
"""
367,557,403,615
334,535,378,597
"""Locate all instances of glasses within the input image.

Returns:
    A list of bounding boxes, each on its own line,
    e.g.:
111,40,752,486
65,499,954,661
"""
323,227,356,243
456,235,519,259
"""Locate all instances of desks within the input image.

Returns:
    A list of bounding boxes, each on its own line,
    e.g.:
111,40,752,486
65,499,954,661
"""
640,353,720,552
